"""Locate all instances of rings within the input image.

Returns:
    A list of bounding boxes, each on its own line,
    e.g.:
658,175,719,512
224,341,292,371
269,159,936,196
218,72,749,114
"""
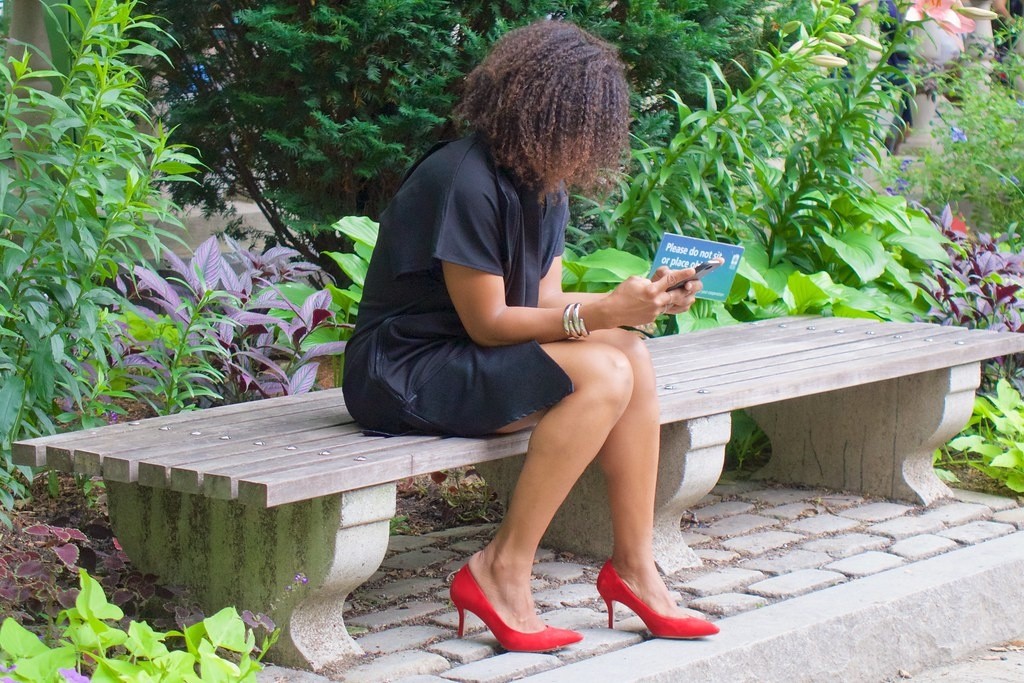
669,292,672,304
663,305,667,314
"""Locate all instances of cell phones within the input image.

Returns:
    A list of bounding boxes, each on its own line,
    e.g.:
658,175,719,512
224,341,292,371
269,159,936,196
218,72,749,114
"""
666,261,719,292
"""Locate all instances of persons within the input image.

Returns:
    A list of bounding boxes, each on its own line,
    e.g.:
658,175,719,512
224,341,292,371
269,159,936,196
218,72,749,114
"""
339,21,720,653
838,0,1024,154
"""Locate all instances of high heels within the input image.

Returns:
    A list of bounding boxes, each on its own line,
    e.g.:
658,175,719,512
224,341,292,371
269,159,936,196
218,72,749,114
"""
450,564,584,652
596,558,720,638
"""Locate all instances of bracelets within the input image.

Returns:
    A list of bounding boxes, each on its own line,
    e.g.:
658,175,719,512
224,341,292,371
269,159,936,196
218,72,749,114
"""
563,302,590,341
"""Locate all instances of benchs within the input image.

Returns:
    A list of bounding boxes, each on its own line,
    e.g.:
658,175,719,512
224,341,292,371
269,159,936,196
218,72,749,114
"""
12,314,1024,672
